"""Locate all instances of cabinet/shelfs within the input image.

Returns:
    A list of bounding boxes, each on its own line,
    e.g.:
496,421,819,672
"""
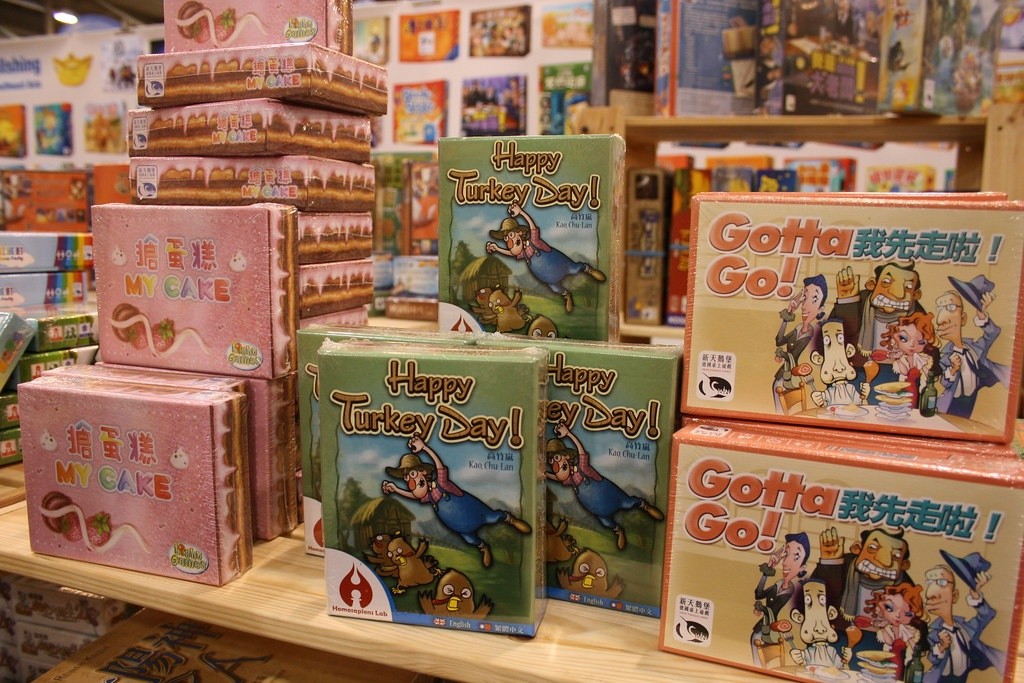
611,106,1022,343
0,485,800,683
